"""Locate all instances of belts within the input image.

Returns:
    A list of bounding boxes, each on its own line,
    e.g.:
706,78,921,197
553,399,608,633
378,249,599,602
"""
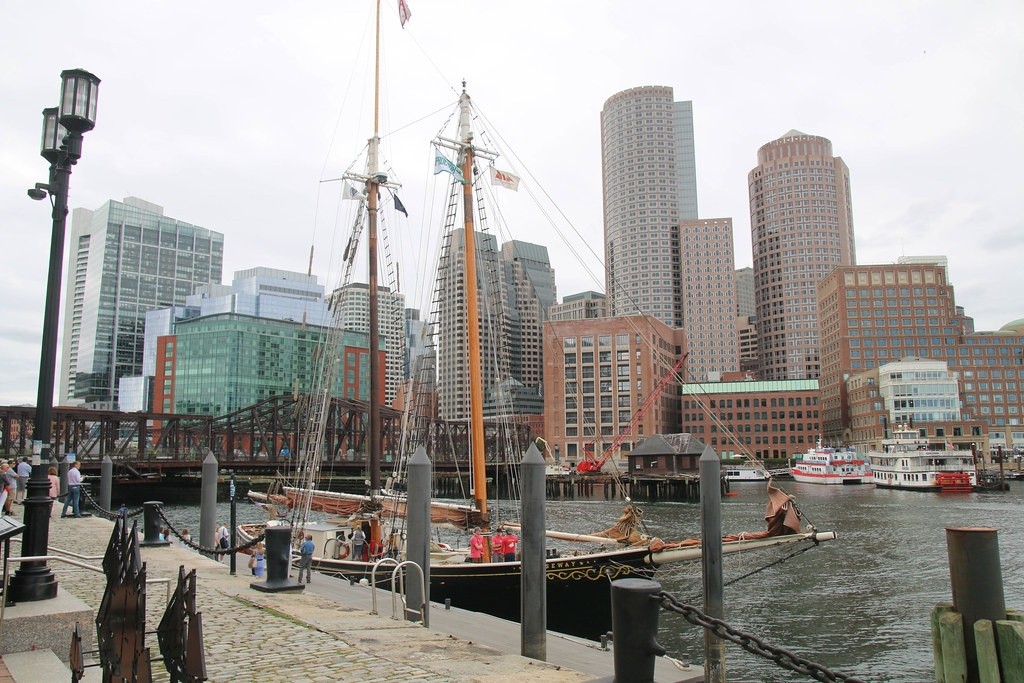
257,559,263,561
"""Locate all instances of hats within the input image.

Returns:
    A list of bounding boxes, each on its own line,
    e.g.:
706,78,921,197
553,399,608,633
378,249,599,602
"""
474,527,482,531
506,527,513,535
497,529,503,533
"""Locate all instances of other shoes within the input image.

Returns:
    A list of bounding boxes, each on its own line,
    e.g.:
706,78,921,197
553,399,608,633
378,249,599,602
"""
5,510,13,516
13,501,21,505
307,580,311,583
61,514,67,518
75,514,82,518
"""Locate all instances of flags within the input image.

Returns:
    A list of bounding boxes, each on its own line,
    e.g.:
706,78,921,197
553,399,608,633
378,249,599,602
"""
394,194,409,217
434,149,465,183
490,166,520,191
342,181,367,200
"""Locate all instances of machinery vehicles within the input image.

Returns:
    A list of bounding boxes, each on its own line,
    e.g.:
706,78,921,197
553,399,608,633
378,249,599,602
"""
575,351,690,474
534,436,576,476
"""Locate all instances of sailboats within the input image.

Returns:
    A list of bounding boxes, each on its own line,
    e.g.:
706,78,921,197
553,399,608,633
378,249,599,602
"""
235,0,841,642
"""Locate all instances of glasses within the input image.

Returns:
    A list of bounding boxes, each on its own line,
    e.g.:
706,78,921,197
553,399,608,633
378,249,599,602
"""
10,463,17,465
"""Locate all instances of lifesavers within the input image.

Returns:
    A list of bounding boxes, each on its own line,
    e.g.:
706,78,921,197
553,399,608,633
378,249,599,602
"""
340,542,350,559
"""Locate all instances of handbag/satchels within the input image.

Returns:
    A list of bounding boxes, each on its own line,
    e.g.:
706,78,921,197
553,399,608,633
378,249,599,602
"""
220,538,228,548
248,549,257,569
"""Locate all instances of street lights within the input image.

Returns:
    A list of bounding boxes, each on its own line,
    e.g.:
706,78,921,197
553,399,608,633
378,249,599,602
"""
5,67,102,604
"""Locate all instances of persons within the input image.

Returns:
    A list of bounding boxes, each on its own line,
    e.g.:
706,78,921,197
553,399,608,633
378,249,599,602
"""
0,457,84,517
298,534,316,584
160,521,230,563
469,527,484,564
491,527,518,563
250,542,266,576
350,525,366,561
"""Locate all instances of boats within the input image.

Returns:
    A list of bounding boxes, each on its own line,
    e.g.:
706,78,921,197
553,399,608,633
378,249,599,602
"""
720,459,770,481
789,433,876,485
866,422,981,492
